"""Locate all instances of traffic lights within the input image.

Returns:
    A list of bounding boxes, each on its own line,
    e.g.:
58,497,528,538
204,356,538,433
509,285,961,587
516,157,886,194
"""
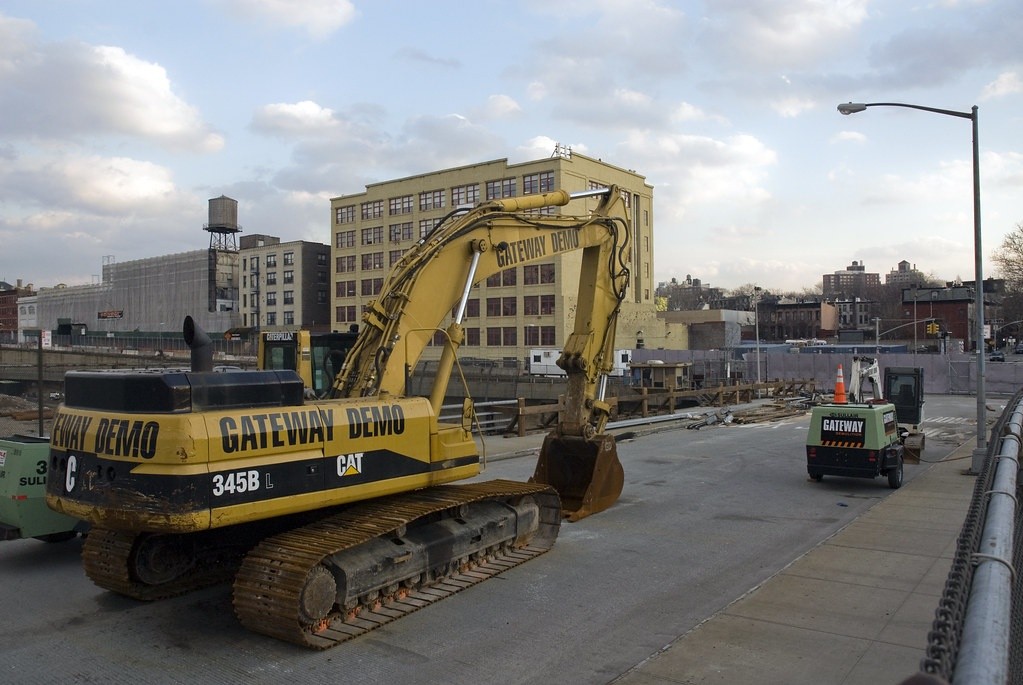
926,323,932,335
932,324,939,335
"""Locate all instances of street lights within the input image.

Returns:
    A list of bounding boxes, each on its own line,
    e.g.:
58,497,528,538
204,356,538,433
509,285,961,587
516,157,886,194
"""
836,101,989,474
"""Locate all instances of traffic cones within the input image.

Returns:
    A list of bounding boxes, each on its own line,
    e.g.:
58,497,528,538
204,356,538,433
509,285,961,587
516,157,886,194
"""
829,363,848,404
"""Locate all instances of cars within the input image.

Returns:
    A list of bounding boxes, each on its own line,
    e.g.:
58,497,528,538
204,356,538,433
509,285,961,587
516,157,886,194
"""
989,352,1004,362
1015,344,1023,354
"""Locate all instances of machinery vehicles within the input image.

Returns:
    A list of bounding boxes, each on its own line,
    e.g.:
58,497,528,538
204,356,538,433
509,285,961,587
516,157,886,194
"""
46,183,640,652
0,433,91,545
805,356,926,489
